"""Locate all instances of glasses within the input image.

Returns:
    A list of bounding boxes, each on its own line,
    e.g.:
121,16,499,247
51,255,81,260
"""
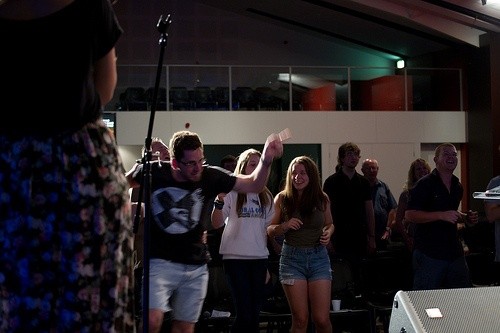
179,158,207,167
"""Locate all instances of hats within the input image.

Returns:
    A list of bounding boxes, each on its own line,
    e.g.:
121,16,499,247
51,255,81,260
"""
0,0,125,70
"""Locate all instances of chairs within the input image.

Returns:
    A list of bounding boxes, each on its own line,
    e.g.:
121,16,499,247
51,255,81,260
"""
302,83,337,111
119,87,302,111
361,75,413,112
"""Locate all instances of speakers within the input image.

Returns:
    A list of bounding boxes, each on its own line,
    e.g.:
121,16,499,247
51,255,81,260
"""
388,285,500,333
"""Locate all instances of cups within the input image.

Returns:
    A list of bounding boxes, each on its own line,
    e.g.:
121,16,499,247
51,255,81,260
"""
332,300,341,311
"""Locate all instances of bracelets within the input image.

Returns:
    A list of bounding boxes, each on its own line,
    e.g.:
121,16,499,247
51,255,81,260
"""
260,157,273,168
213,198,225,210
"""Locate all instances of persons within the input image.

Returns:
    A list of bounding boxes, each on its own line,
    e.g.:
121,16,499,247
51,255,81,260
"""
323,143,376,310
267,156,333,333
395,159,431,289
211,148,276,333
405,143,479,290
483,176,500,286
361,159,398,310
221,155,237,173
130,134,170,333
126,128,291,333
0,0,136,333
